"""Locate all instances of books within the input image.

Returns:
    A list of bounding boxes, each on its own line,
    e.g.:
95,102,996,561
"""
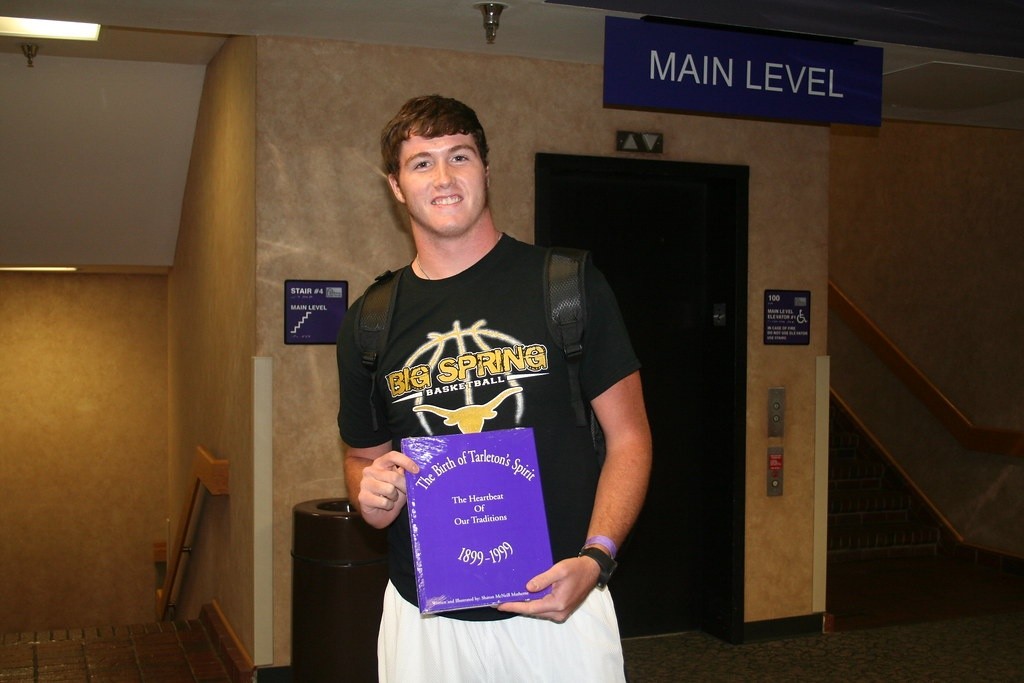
401,426,556,616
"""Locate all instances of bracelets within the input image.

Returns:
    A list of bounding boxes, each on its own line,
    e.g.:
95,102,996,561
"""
583,536,617,559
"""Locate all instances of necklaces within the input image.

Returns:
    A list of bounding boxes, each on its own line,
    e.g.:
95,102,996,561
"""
415,233,503,279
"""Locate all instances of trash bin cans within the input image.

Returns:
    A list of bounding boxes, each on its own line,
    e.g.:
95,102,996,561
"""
291,499,389,683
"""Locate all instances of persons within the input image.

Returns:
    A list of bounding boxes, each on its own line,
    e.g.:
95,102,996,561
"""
337,94,656,683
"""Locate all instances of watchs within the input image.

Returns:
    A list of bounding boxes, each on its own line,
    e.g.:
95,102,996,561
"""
578,548,618,588
